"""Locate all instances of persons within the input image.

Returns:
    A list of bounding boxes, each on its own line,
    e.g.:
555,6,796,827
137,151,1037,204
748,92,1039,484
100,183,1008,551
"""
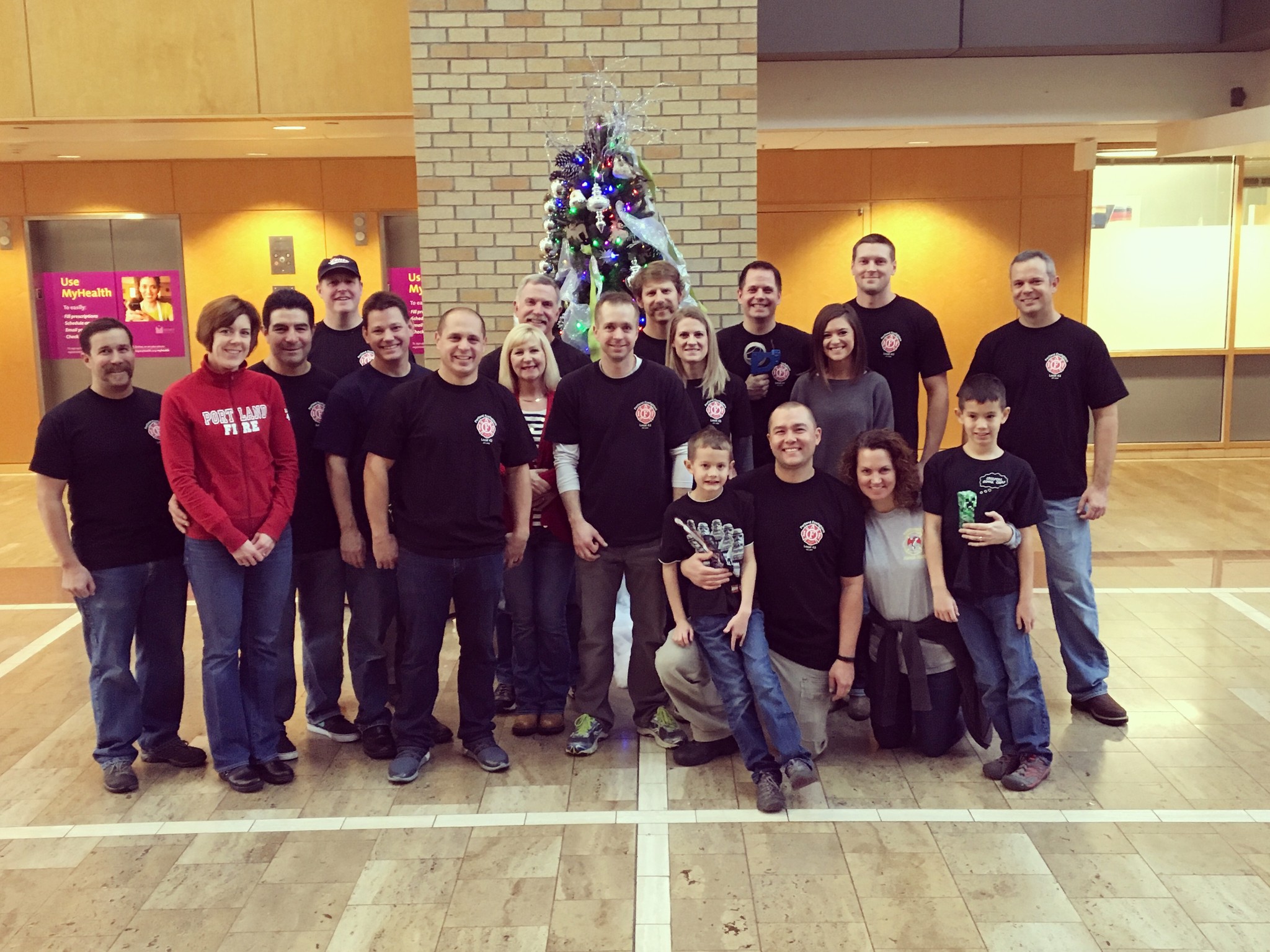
320,291,454,762
633,261,695,723
28,318,209,797
497,321,581,738
665,306,761,488
837,426,1021,763
919,372,1055,793
656,425,820,815
478,272,583,718
125,276,174,321
167,288,364,764
305,254,400,708
657,399,866,768
812,233,953,510
161,295,300,796
543,290,697,760
363,306,539,787
715,260,815,472
788,302,895,486
956,249,1131,728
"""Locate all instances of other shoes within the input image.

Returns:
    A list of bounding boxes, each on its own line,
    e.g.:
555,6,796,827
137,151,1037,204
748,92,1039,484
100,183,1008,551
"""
846,695,871,721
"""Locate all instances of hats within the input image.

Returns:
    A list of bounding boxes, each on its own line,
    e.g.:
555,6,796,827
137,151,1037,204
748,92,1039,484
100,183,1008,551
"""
318,255,361,282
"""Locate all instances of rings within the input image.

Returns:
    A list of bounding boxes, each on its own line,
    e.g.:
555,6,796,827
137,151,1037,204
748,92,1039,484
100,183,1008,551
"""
579,554,584,557
263,553,266,558
979,536,982,541
242,561,248,565
514,557,519,564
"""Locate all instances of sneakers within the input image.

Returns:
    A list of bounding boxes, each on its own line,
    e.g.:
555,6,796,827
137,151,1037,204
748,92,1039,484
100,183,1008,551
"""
387,745,431,782
306,714,360,742
361,724,397,760
565,714,610,756
277,730,298,761
1001,753,1051,791
755,772,786,812
426,714,454,744
141,740,207,768
103,761,140,794
785,758,818,791
672,735,740,767
983,754,1020,780
459,736,511,770
494,683,518,712
636,705,688,749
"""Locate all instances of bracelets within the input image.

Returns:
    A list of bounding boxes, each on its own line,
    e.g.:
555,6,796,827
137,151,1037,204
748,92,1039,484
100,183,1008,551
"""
835,655,857,663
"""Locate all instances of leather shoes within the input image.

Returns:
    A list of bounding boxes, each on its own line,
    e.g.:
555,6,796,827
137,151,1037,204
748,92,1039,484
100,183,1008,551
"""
218,765,265,793
1071,693,1129,725
254,756,295,785
511,711,565,737
